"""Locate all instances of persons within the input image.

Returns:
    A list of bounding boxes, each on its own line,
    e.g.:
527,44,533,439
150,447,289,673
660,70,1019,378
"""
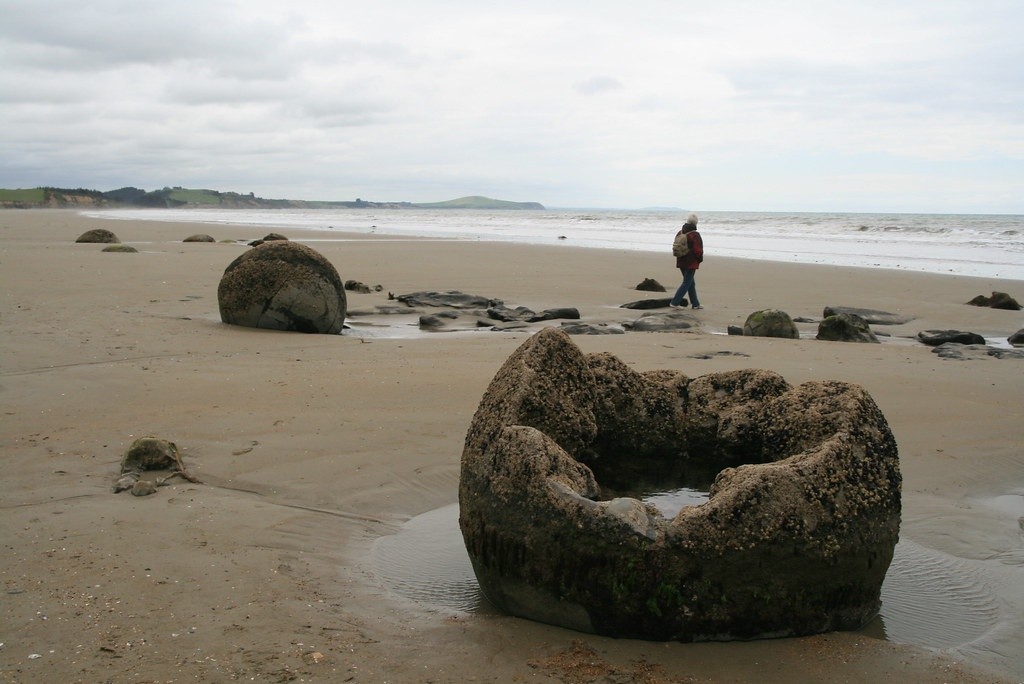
669,213,704,310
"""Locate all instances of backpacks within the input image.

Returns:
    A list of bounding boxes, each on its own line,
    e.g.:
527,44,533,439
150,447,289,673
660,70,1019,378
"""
672,230,696,257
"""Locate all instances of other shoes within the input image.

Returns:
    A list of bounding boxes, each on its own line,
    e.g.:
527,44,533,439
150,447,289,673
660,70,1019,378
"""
692,305,704,309
669,303,689,309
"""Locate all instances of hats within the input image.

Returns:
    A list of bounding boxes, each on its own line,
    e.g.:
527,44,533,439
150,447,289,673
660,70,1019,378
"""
686,213,698,224
